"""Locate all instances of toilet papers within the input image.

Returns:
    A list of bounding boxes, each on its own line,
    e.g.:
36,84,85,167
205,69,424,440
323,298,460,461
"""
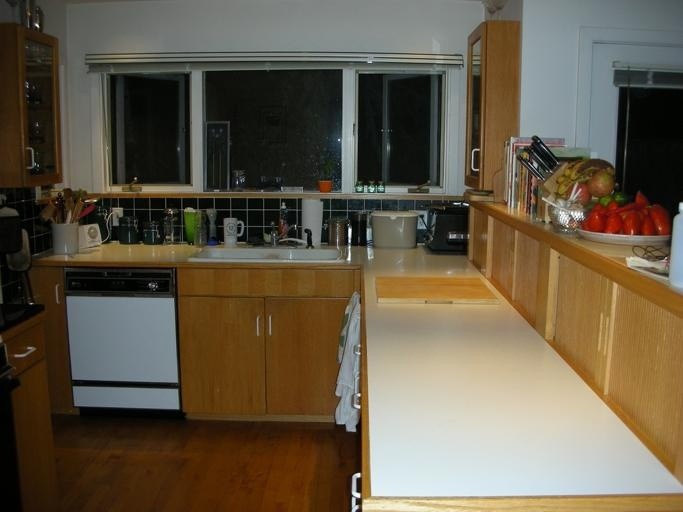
302,198,324,245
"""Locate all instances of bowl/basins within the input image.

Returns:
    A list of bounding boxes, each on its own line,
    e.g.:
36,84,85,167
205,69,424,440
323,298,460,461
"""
546,200,589,233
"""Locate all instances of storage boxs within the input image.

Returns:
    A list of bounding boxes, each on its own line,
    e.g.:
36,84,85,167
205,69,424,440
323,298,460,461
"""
369,211,418,248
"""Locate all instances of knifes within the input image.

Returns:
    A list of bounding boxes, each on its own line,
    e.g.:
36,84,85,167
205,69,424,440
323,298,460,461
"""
516,133,561,182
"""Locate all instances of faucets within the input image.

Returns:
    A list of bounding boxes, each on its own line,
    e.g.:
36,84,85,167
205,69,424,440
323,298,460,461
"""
271,223,298,244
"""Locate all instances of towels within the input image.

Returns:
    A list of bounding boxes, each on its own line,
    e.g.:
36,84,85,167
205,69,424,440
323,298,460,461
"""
337,291,360,363
334,302,361,433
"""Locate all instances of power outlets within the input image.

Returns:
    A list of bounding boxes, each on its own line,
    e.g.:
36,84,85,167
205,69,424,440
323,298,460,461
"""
410,210,427,229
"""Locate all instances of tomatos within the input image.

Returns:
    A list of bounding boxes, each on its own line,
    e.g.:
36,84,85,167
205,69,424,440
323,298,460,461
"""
581,191,671,235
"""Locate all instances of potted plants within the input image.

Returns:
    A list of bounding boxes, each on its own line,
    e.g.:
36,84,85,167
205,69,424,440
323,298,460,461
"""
318,159,336,193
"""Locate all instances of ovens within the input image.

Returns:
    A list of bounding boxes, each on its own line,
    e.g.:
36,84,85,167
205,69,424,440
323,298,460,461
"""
0,341,25,512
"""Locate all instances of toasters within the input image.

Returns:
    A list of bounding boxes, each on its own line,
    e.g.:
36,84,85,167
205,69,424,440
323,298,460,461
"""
426,205,469,256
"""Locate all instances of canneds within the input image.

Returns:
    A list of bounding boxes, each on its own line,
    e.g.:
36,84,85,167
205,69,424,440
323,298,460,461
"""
367,180,376,193
377,180,385,193
355,181,364,193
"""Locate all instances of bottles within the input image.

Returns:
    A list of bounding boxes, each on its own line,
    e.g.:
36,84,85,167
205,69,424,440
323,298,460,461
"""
118,207,219,247
327,215,350,246
354,180,386,193
666,200,683,289
19,0,43,34
263,201,291,249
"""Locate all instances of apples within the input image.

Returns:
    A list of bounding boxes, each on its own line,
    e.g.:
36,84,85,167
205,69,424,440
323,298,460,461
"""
587,171,615,197
566,183,592,204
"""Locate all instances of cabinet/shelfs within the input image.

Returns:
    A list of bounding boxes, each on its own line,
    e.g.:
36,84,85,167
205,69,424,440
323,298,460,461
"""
177,269,355,422
464,20,520,192
491,218,541,323
28,266,80,416
4,310,62,512
473,209,485,267
554,254,683,485
0,23,62,189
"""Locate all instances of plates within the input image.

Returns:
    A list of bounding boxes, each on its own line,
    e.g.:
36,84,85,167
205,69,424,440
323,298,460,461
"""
572,225,671,248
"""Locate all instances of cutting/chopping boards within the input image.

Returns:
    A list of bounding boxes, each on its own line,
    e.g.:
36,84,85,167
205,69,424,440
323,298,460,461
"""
374,275,499,305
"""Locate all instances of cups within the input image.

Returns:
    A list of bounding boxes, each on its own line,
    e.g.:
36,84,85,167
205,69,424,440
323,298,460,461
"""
21,40,55,179
230,168,282,193
223,217,245,245
52,221,77,255
318,181,333,193
349,212,367,248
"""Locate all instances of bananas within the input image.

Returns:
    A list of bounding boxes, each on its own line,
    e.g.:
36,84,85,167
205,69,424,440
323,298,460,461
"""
556,158,615,199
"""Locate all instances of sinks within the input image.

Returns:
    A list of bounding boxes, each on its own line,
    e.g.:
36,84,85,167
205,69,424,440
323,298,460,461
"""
187,247,341,265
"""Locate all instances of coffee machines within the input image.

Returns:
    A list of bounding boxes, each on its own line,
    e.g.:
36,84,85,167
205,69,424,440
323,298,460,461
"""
0,193,44,335
